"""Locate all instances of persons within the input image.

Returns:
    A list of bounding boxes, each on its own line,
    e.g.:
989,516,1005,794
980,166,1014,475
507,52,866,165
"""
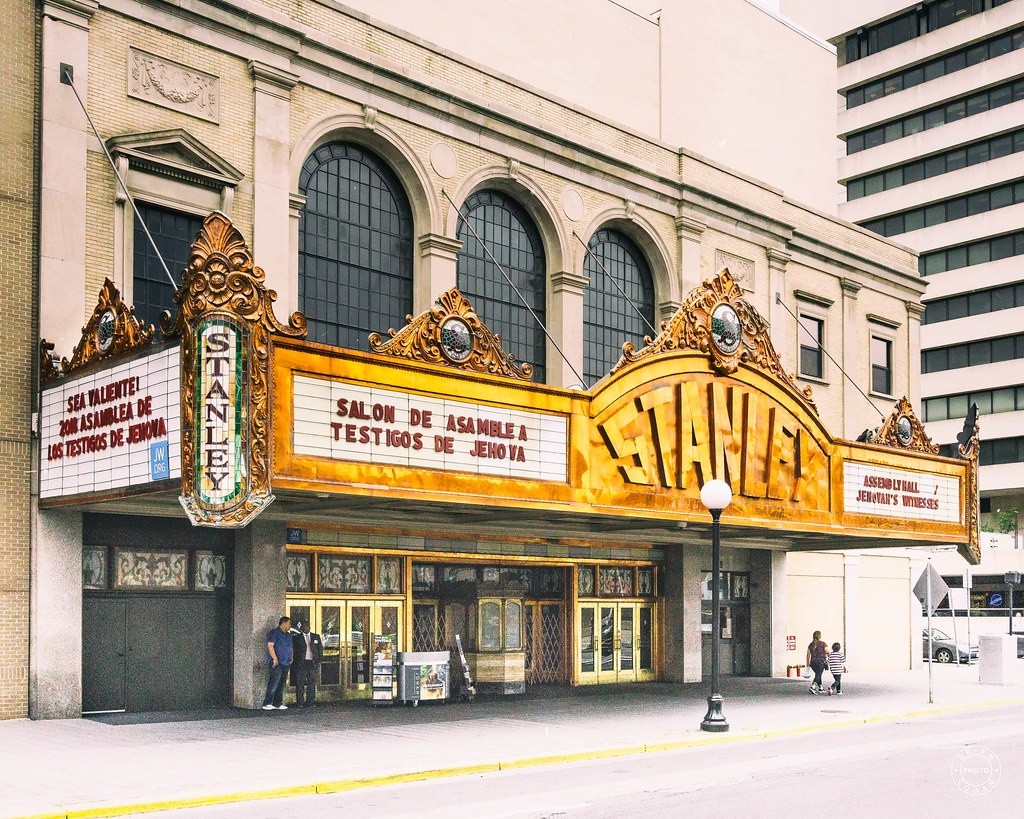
827,643,847,696
806,630,830,694
261,617,293,710
293,622,323,708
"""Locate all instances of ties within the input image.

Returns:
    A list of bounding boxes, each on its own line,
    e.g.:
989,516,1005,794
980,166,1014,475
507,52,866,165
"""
305,634,311,660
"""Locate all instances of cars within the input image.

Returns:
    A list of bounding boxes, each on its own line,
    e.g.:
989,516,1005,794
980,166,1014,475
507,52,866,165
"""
923,628,979,664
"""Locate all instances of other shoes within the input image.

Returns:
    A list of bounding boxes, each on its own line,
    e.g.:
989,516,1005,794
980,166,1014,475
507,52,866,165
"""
278,705,288,709
818,688,827,693
837,690,844,694
263,705,276,710
828,686,833,696
810,687,817,695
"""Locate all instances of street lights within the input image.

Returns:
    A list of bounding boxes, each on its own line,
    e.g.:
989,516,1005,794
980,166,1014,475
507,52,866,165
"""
700,478,733,733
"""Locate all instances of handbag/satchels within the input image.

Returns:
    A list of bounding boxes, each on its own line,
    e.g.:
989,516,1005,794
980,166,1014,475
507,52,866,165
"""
803,666,811,678
824,661,829,670
